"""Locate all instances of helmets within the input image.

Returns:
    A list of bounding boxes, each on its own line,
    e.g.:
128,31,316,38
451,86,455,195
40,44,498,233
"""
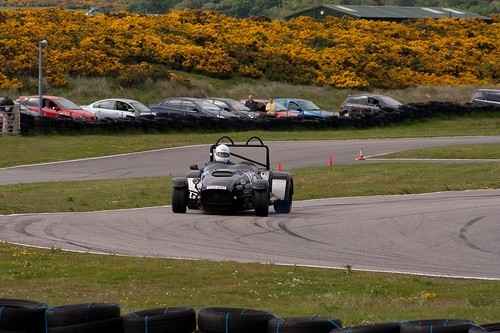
214,144,230,163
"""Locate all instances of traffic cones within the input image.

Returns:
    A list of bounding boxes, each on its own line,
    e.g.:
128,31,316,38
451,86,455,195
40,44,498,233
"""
276,163,281,170
358,148,365,160
327,154,333,166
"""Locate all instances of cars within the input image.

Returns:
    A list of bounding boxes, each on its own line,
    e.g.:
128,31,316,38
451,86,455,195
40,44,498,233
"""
0,98,41,132
148,98,262,121
239,99,340,121
172,136,294,217
14,96,101,123
79,99,159,118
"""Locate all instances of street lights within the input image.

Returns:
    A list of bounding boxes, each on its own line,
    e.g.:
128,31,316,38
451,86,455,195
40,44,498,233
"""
39,40,48,118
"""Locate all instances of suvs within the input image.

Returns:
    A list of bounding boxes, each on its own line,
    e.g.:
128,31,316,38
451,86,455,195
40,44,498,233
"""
340,95,416,119
471,88,500,107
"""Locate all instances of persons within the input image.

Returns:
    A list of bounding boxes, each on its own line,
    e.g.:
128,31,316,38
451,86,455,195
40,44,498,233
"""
368,98,376,106
245,95,257,112
265,98,276,115
118,102,124,110
44,102,52,109
214,144,235,165
0,96,14,132
